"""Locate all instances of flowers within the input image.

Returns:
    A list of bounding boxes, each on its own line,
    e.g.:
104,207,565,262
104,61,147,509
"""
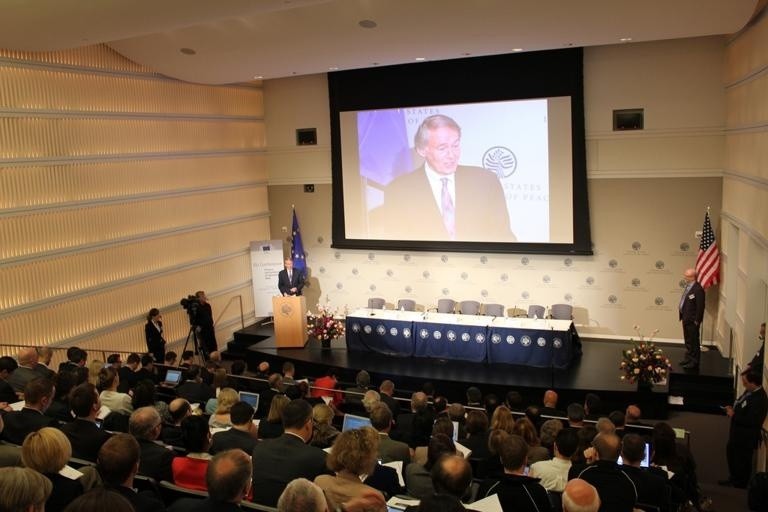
619,326,671,386
305,305,345,341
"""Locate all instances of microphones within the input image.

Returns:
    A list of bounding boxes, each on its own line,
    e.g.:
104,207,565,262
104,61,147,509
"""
369,299,376,316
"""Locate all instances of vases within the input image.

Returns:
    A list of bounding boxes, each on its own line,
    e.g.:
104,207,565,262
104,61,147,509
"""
321,338,330,350
637,380,652,392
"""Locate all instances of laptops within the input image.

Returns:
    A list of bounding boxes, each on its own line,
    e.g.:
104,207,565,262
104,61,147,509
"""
95,418,105,430
342,412,372,434
432,420,459,441
617,441,650,469
161,369,182,389
239,390,260,417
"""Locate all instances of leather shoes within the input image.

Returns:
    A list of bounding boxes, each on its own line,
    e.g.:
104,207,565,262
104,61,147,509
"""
718,476,732,488
679,360,689,370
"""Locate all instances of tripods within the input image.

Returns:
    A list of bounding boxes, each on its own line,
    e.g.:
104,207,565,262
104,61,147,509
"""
176,326,213,368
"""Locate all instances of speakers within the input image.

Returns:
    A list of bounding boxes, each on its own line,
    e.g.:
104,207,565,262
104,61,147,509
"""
612,108,645,133
296,127,317,146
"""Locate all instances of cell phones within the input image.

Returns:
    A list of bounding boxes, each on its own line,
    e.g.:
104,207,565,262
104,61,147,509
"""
524,464,530,476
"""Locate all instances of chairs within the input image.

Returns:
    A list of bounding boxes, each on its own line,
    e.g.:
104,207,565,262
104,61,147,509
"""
369,297,575,367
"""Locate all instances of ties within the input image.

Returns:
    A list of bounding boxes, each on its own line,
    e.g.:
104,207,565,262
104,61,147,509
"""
441,178,454,236
289,271,292,285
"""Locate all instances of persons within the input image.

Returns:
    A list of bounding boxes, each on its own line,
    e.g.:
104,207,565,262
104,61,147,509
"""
747,322,766,382
1,347,694,511
143,307,168,362
717,367,768,490
381,114,518,241
676,267,705,369
192,291,220,360
276,256,305,296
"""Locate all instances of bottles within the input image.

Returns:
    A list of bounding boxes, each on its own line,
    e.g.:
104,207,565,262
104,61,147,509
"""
533,309,538,326
401,306,405,318
382,305,386,317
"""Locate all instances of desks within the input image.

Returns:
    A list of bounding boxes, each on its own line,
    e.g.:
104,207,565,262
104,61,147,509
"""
347,309,574,369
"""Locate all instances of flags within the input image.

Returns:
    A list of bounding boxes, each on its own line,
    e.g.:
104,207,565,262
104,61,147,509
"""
290,203,308,280
695,209,720,290
